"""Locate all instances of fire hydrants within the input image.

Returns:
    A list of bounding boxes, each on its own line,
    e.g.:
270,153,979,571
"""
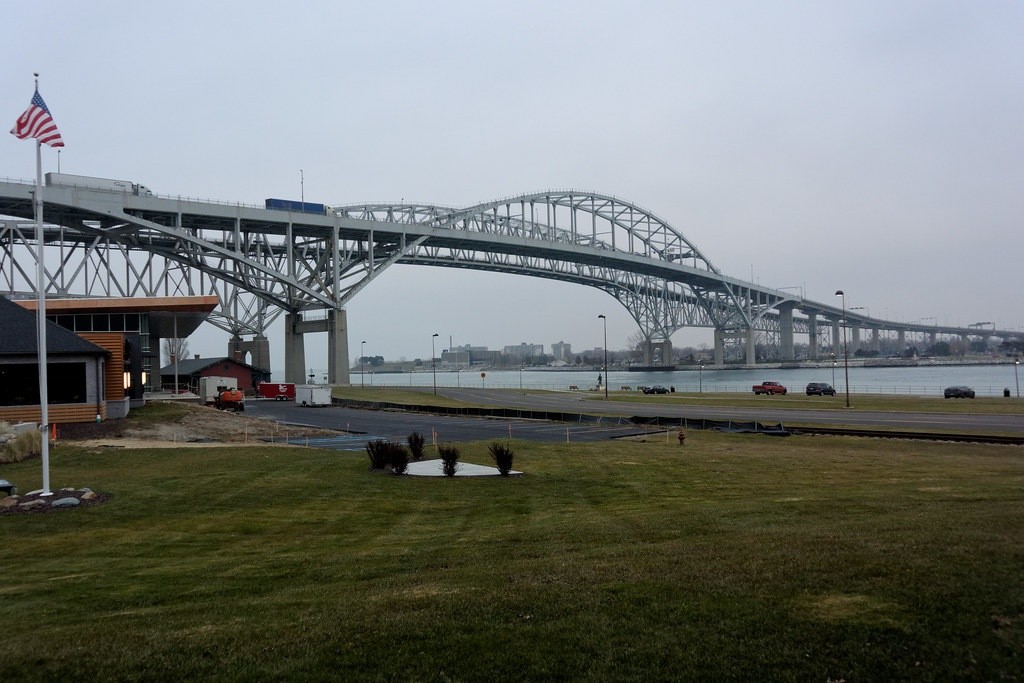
677,430,686,446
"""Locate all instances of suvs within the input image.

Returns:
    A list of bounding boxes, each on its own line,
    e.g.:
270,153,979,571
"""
806,382,837,397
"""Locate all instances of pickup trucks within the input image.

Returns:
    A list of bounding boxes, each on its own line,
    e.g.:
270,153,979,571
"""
752,381,788,396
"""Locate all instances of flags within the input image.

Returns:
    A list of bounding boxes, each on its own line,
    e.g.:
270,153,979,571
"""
10,89,65,147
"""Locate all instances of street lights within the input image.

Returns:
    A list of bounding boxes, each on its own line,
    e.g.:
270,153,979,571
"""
458,367,462,387
1014,358,1019,397
361,340,366,389
401,198,403,224
371,371,374,386
409,367,414,386
57,149,61,173
834,290,850,407
432,333,439,394
597,314,607,398
832,360,837,389
699,359,705,393
519,364,525,389
300,170,305,213
598,362,603,385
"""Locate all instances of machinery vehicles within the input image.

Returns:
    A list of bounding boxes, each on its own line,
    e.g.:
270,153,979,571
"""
212,386,245,412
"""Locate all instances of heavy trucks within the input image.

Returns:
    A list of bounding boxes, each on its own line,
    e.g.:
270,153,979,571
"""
265,198,336,216
45,171,152,197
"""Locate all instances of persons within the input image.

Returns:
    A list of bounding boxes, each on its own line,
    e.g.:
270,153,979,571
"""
598,373,602,385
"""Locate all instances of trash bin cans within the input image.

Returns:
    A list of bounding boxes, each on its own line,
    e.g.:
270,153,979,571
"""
671,385,675,392
1004,388,1010,397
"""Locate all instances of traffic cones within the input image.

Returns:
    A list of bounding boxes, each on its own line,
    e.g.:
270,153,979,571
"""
51,423,57,439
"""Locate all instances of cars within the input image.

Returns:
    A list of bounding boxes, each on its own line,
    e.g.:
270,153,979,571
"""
643,385,669,395
944,385,976,399
